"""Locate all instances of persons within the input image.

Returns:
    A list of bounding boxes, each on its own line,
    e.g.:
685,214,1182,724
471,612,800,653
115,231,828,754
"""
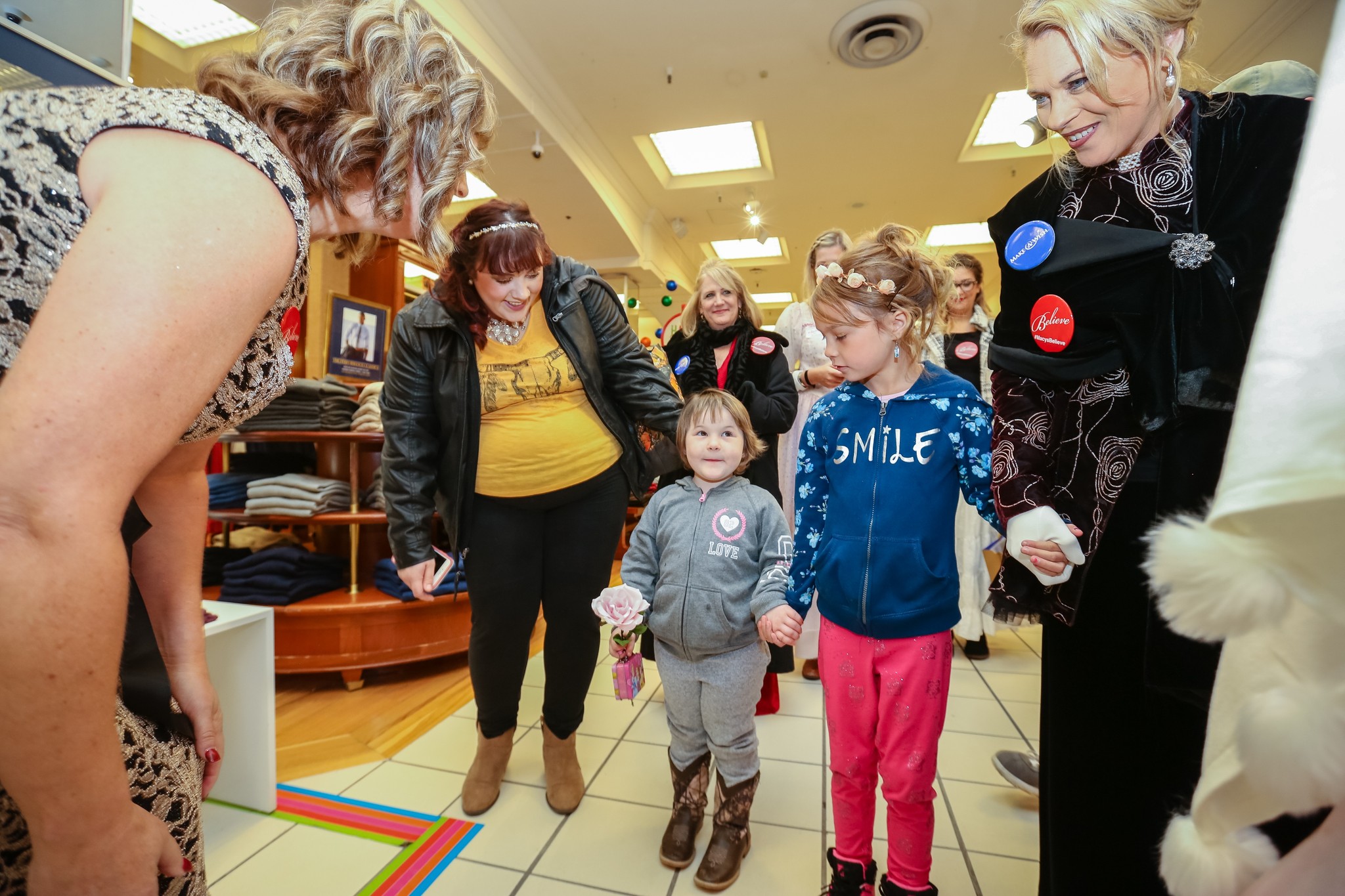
757,221,1085,895
640,255,803,717
770,229,856,679
991,59,1317,799
986,1,1316,894
0,0,499,895
608,389,803,891
381,197,688,816
339,309,371,362
909,252,1000,659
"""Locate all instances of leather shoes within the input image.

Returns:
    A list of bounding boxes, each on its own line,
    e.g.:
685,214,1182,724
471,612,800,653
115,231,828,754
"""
964,630,990,659
801,659,820,680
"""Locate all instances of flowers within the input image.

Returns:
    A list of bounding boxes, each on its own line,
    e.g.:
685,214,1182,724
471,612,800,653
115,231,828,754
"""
592,583,651,647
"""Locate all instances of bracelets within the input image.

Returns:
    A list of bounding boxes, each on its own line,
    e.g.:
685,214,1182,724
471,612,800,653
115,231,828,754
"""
798,369,816,389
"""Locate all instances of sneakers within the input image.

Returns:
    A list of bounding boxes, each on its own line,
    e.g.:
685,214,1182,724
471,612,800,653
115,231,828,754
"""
991,749,1039,797
878,873,938,896
820,848,876,896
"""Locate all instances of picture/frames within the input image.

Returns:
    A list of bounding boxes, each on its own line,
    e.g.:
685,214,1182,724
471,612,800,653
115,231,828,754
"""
322,289,392,385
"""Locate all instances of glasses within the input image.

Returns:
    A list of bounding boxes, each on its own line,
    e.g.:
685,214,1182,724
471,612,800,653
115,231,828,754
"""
954,281,978,291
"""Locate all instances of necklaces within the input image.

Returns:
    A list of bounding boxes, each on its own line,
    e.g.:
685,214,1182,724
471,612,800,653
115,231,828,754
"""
1115,95,1188,170
479,305,526,346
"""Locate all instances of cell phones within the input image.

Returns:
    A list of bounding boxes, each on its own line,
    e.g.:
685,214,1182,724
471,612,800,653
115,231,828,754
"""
391,544,454,591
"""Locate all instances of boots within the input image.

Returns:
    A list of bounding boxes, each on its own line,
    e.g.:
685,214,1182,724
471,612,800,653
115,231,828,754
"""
460,721,517,818
540,716,585,815
660,746,711,868
693,768,760,892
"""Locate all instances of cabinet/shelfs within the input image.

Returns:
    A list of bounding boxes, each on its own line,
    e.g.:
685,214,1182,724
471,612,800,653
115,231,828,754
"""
208,428,472,694
